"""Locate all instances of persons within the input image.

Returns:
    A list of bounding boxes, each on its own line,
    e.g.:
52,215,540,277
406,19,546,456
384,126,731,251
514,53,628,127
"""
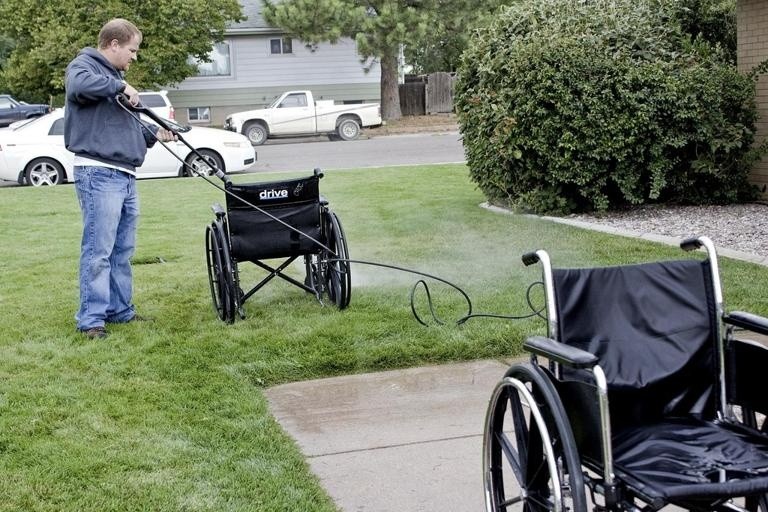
64,18,178,339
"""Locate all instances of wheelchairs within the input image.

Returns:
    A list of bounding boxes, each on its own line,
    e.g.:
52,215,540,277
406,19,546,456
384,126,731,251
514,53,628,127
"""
203,164,353,328
475,229,766,512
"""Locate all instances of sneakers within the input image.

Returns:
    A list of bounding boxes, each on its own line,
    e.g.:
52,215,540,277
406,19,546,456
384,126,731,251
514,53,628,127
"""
124,313,155,323
76,327,107,339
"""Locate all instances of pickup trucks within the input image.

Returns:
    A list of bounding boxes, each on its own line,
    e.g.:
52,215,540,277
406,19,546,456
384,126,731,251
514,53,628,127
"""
222,90,387,149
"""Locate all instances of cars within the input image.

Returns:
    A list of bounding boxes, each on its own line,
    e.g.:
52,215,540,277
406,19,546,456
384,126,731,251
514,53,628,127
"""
0,89,257,188
